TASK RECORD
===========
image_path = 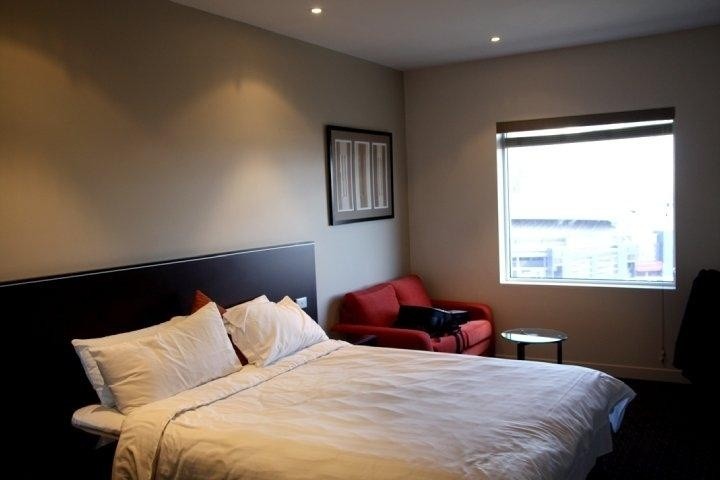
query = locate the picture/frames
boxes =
[324,123,394,227]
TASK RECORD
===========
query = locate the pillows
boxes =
[88,301,243,418]
[223,293,273,366]
[72,314,188,408]
[190,287,248,367]
[221,296,329,366]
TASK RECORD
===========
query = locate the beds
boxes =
[0,240,638,479]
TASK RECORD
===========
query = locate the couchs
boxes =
[330,272,497,357]
